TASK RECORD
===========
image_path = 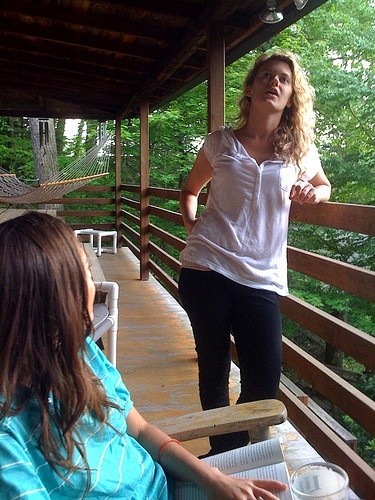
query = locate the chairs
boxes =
[91,281,119,369]
[148,398,287,444]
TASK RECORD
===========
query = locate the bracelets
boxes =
[158,439,181,465]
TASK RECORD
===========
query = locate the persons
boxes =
[177,47,331,458]
[0,211,285,500]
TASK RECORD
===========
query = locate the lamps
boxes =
[259,0,283,24]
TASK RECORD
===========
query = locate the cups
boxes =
[290,462,349,500]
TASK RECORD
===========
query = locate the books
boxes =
[174,438,294,500]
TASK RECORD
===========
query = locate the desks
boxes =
[74,228,117,256]
